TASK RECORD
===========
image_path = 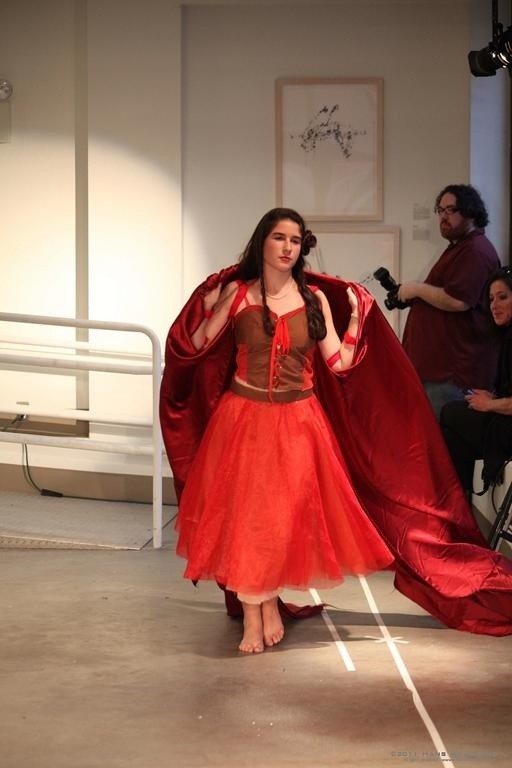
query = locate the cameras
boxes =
[372,266,414,310]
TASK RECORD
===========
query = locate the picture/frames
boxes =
[304,222,401,339]
[274,76,384,223]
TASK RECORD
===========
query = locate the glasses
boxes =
[434,206,459,216]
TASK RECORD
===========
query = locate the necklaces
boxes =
[258,278,294,299]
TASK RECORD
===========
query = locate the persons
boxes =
[395,182,503,425]
[171,209,396,655]
[437,267,510,512]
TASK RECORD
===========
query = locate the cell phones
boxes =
[461,386,476,396]
